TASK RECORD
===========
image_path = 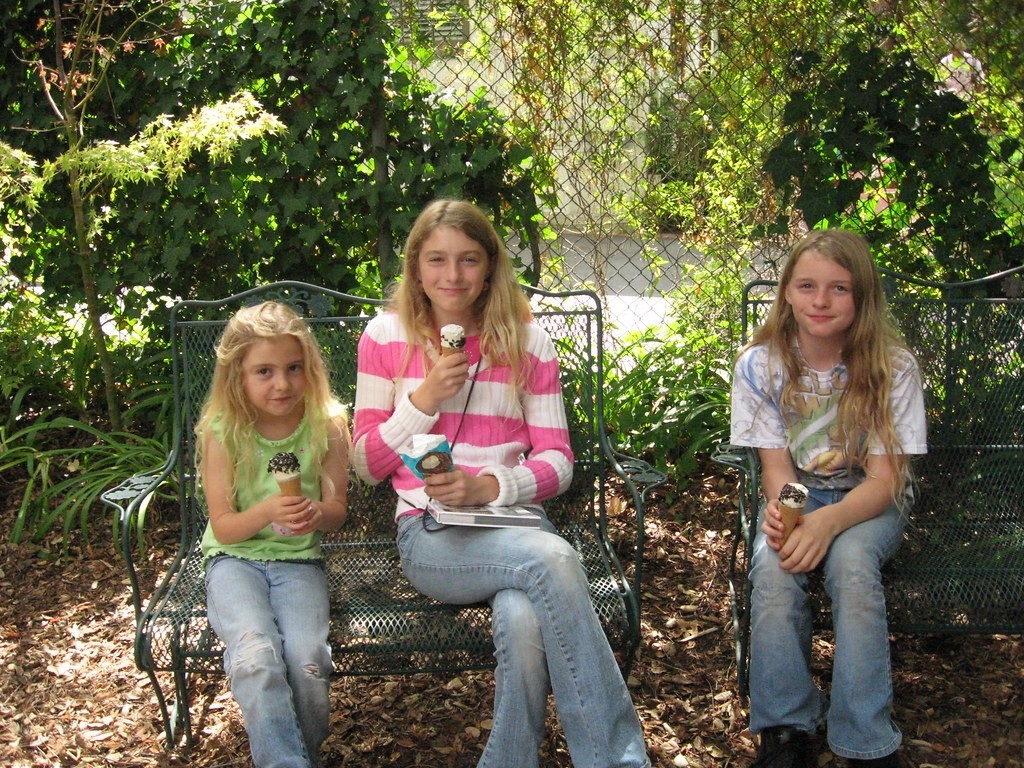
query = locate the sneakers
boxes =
[746,726,902,768]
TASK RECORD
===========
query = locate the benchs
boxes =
[727,267,1023,700]
[123,276,649,749]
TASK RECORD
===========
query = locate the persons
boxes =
[352,201,657,768]
[729,231,932,767]
[196,301,352,767]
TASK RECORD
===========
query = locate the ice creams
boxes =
[414,451,451,478]
[775,482,809,548]
[439,324,465,358]
[266,452,301,497]
